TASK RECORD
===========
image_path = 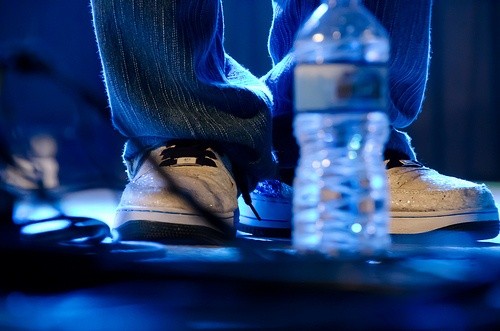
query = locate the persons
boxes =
[92,0,500,246]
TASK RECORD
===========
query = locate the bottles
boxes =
[291,0,393,261]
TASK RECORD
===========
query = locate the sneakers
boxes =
[111,137,263,246]
[236,125,500,244]
